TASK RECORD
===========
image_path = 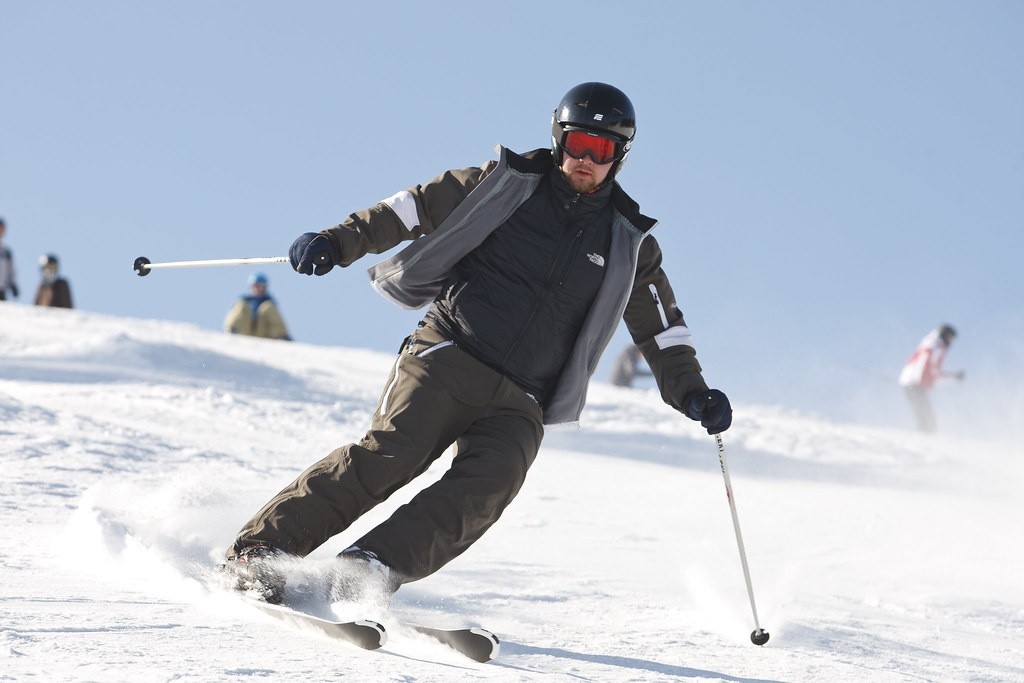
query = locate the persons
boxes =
[899,322,965,432]
[34,254,72,308]
[217,81,732,608]
[612,343,653,387]
[0,220,19,302]
[223,272,291,340]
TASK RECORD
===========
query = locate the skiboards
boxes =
[242,596,500,668]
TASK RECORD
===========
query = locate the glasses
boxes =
[559,127,627,165]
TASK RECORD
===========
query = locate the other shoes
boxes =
[218,544,286,604]
[323,546,403,616]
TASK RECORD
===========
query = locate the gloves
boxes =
[686,388,732,435]
[289,233,340,275]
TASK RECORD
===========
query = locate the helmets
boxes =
[38,254,58,271]
[551,82,637,179]
[249,273,267,284]
